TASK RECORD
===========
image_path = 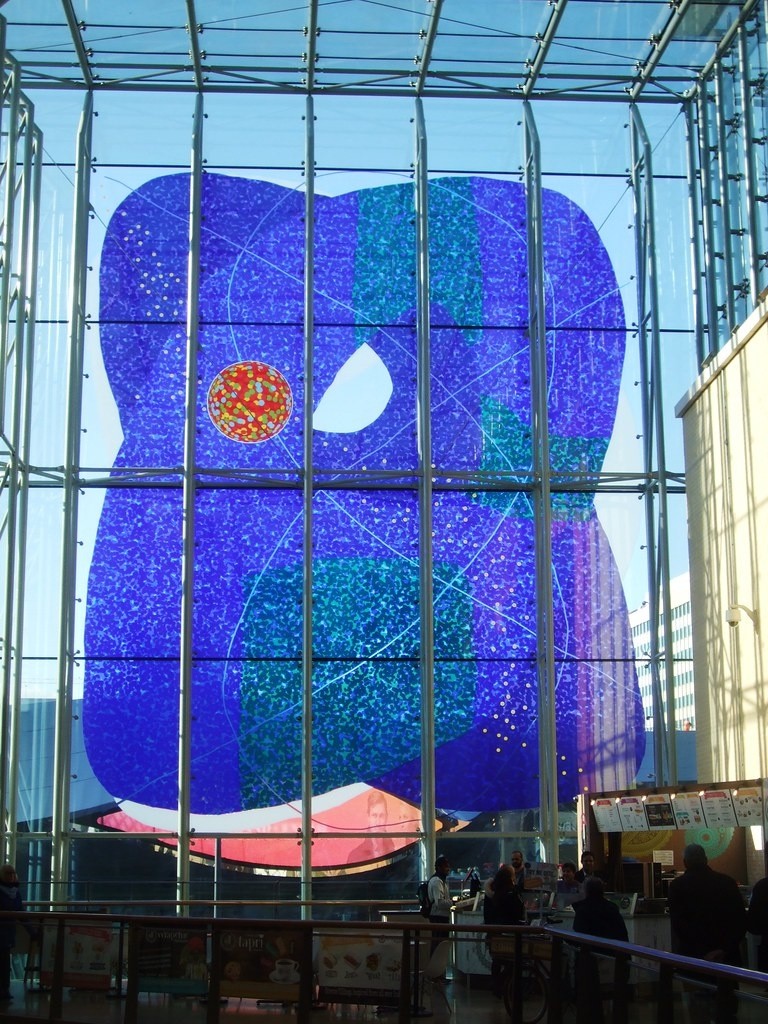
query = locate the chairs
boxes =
[410,940,453,1013]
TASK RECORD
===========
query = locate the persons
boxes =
[484,851,528,1001]
[557,851,633,1024]
[668,845,768,1023]
[1,867,38,1004]
[428,859,452,983]
[346,791,419,864]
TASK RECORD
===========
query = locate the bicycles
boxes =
[501,913,612,1023]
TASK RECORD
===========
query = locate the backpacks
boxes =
[417,875,443,918]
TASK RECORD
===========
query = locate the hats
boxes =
[435,857,448,866]
